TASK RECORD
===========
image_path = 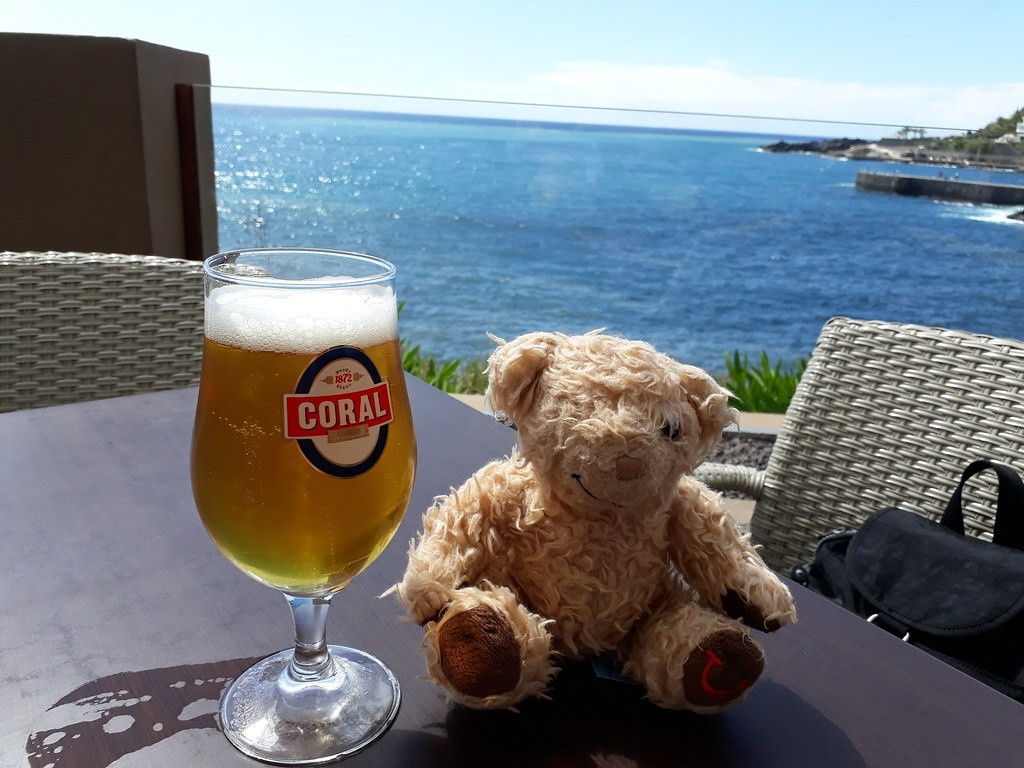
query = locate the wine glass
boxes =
[188,247,419,765]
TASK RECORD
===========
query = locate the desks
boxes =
[0,369,1024,768]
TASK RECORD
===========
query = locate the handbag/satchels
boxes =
[792,461,1024,704]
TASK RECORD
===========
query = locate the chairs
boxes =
[683,314,1024,579]
[0,252,270,414]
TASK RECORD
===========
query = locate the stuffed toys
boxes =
[394,327,799,716]
